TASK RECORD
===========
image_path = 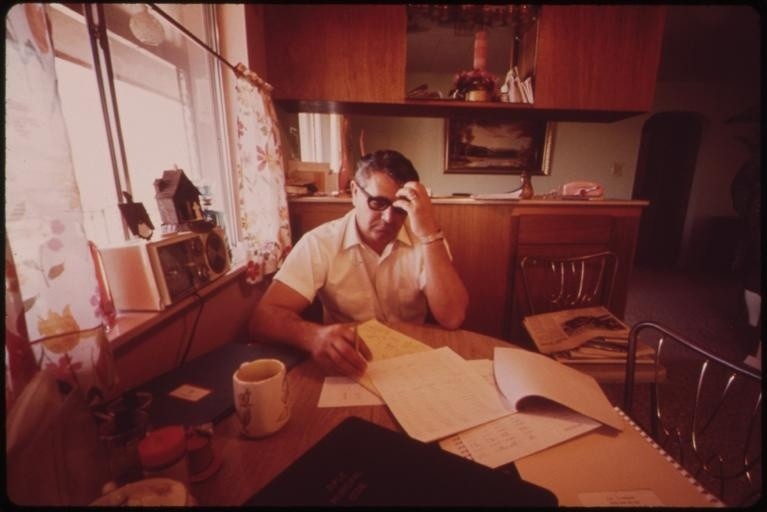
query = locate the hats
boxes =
[137,423,224,483]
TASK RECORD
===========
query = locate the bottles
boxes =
[520,168,534,199]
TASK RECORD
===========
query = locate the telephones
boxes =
[563,181,604,197]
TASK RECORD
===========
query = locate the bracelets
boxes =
[419,230,444,244]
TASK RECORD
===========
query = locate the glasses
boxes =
[354,181,407,215]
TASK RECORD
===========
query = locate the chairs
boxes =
[626,321,761,506]
[519,250,668,445]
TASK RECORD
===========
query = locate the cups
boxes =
[233,357,294,439]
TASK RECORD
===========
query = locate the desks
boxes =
[22,317,730,508]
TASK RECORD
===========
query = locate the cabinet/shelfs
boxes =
[705,217,752,276]
[282,191,651,342]
[254,5,664,113]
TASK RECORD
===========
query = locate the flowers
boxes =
[454,69,498,90]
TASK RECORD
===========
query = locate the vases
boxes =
[465,85,487,102]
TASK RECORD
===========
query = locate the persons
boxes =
[246,151,469,378]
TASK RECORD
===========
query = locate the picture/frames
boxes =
[441,118,558,176]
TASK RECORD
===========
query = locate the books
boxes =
[470,189,522,199]
[284,180,317,194]
[513,407,725,508]
[317,307,665,470]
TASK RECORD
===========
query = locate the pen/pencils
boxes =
[352,322,359,352]
